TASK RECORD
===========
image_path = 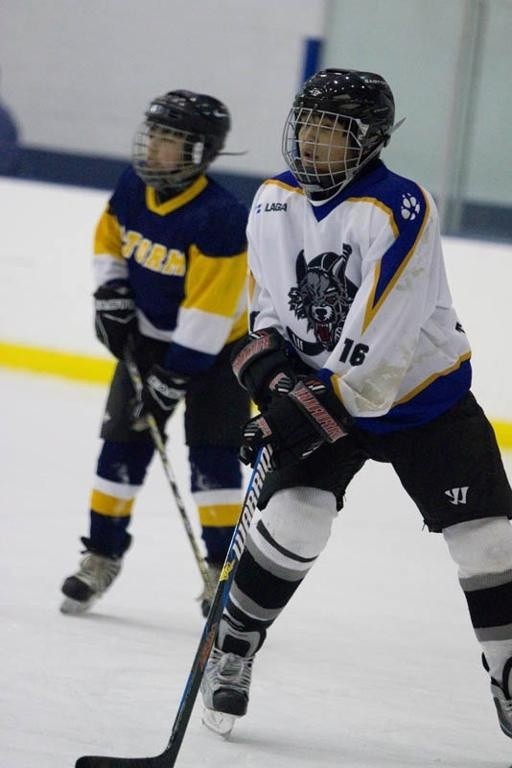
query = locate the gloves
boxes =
[94,284,140,358]
[229,326,349,468]
[125,366,190,443]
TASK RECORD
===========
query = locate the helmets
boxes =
[148,90,229,162]
[295,69,394,145]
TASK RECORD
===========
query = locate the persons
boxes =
[199,68,511,738]
[60,90,256,615]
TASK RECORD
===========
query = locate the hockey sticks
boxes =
[76,444,278,767]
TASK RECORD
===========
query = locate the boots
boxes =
[63,531,132,602]
[202,555,265,714]
[482,652,511,738]
